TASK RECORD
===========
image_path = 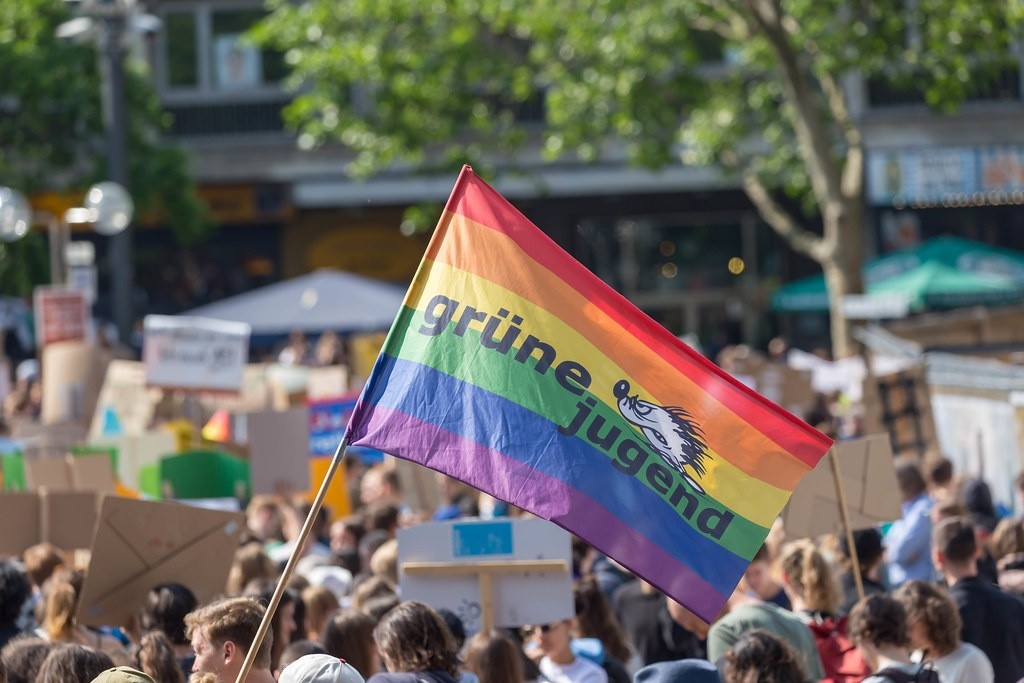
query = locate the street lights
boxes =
[54,9,164,355]
[0,180,133,288]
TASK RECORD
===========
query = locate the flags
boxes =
[345,163,838,626]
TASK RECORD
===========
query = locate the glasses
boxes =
[523,622,563,636]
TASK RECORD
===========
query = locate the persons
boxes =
[266,330,352,368]
[4,360,43,435]
[440,525,709,682]
[719,632,818,683]
[366,599,480,683]
[7,470,405,682]
[663,593,828,681]
[739,460,1023,683]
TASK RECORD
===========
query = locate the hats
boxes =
[91,666,154,683]
[279,654,365,682]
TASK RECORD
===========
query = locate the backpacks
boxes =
[878,667,940,682]
[799,618,871,683]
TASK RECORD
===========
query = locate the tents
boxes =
[774,234,1023,321]
[175,267,414,338]
